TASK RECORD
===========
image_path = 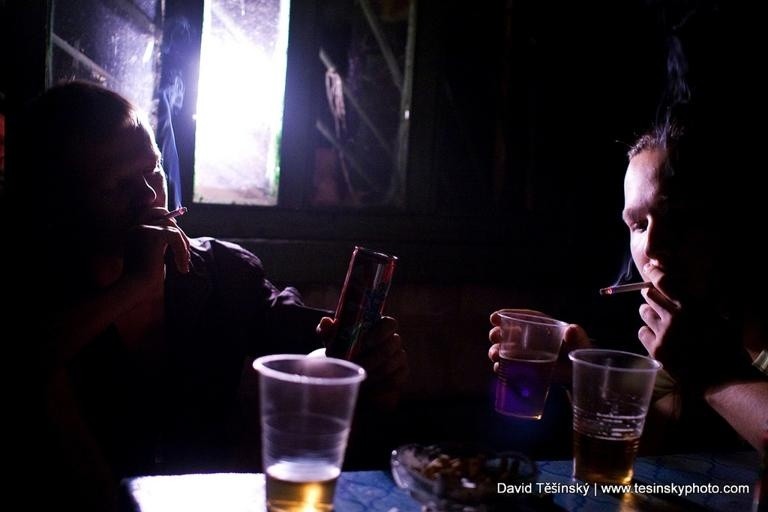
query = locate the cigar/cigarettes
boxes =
[599,281,653,295]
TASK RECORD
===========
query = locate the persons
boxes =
[0,81,410,512]
[159,207,187,219]
[487,124,768,457]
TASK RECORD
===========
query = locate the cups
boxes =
[495,311,663,488]
[253,354,368,512]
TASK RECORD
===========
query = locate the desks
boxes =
[118,453,760,512]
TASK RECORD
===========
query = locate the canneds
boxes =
[325,245,400,364]
